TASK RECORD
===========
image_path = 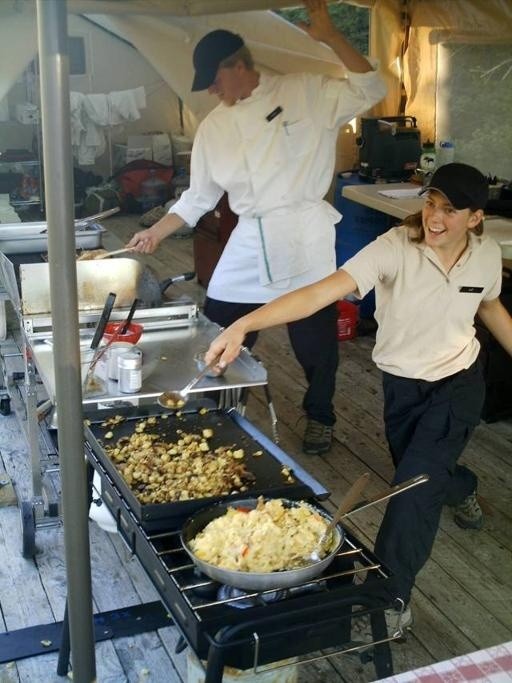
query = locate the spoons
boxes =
[285,473,372,572]
[156,349,223,409]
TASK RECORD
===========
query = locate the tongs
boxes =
[73,206,122,232]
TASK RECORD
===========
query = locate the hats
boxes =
[190,28,244,93]
[417,161,489,212]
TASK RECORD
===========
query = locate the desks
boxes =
[342,181,512,274]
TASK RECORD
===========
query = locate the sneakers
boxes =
[335,602,414,654]
[450,481,481,533]
[297,414,332,456]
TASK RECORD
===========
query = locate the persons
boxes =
[123,1,388,456]
[204,162,512,658]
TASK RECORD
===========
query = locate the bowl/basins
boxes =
[193,351,226,378]
[180,473,431,590]
[103,321,143,346]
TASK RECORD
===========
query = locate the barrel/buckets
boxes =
[335,300,361,342]
